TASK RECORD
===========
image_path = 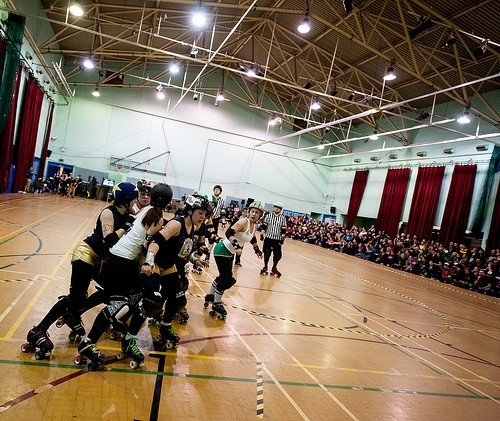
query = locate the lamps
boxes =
[341,0,353,12]
[91,88,100,97]
[446,34,456,46]
[310,95,321,110]
[457,108,471,124]
[98,69,103,76]
[382,63,397,82]
[297,14,311,34]
[302,80,355,101]
[118,73,123,80]
[369,128,380,141]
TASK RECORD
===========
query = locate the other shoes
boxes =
[234,261,242,267]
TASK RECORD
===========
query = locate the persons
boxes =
[23,166,99,199]
[108,179,264,369]
[72,183,173,371]
[260,203,286,277]
[22,182,138,360]
[284,213,500,298]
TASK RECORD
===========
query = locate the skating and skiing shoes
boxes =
[74,337,106,371]
[173,308,190,324]
[270,267,282,278]
[116,337,145,369]
[55,311,86,341]
[260,267,269,274]
[191,265,203,275]
[147,311,164,329]
[209,301,227,321]
[106,319,129,341]
[20,325,54,360]
[155,323,181,350]
[204,294,215,308]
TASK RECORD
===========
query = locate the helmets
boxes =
[112,182,139,199]
[151,183,173,203]
[213,185,222,192]
[186,194,210,211]
[136,179,153,193]
[274,202,283,210]
[248,201,265,217]
